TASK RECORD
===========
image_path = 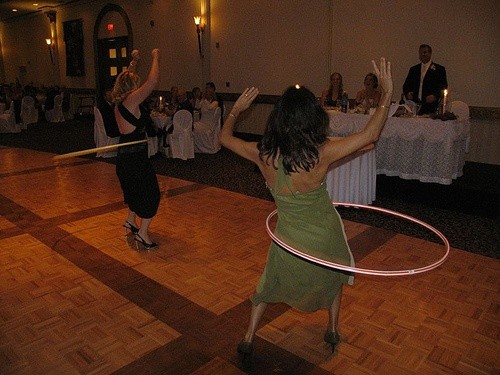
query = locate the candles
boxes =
[443,90,447,114]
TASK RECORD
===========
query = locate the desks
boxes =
[323,136,377,205]
[327,110,468,185]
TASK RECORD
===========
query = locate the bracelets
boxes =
[377,104,390,109]
[228,113,238,120]
[129,61,137,67]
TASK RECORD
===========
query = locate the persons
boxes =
[220,57,393,361]
[98,82,218,158]
[402,44,447,115]
[321,72,382,110]
[0,82,69,123]
[111,49,160,248]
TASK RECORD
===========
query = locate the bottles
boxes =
[342,92,348,112]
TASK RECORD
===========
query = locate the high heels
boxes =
[122,221,140,235]
[133,233,159,253]
[324,331,339,353]
[237,340,253,363]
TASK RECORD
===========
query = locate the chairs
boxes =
[74,96,95,146]
[146,135,158,158]
[449,102,469,118]
[0,92,76,133]
[194,107,221,155]
[93,105,120,158]
[166,110,196,161]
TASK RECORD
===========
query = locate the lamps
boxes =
[193,16,202,26]
[45,38,52,45]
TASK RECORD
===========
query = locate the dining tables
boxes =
[150,111,200,148]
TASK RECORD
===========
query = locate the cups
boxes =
[336,100,342,111]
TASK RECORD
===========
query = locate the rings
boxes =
[245,94,249,97]
